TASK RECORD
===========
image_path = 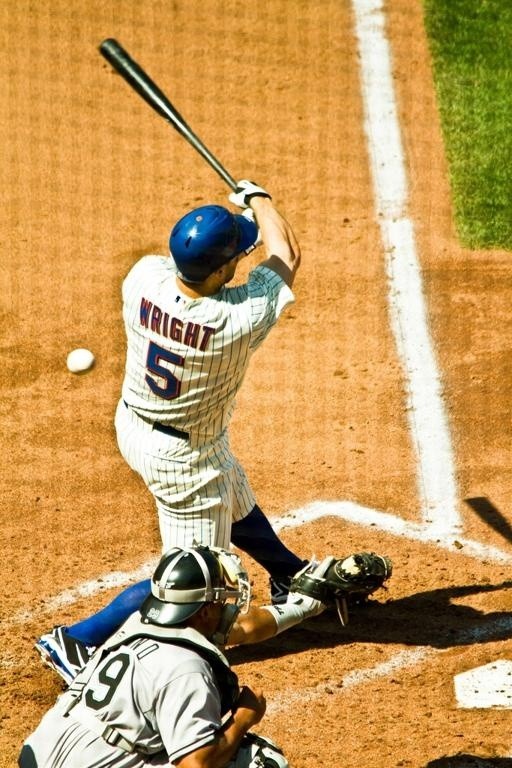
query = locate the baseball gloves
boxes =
[289,551,393,622]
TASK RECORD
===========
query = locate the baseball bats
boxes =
[98,38,242,194]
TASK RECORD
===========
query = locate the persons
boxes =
[34,179,315,686]
[14,540,289,768]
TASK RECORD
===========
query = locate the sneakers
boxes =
[269,560,309,605]
[33,624,97,691]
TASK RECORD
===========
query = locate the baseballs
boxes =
[67,350,94,374]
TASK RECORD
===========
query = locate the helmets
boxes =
[169,204,257,281]
[139,544,252,650]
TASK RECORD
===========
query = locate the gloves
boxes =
[259,556,336,638]
[229,180,271,255]
